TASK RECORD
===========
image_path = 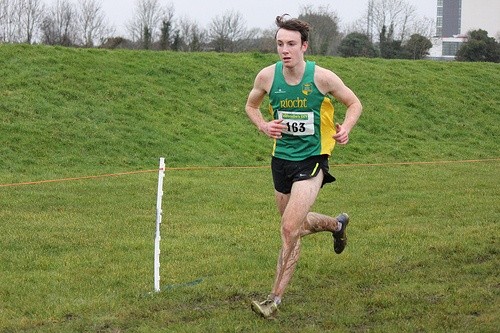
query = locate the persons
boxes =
[246,13,365,318]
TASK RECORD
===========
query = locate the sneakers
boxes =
[333,213,350,254]
[251,300,280,319]
